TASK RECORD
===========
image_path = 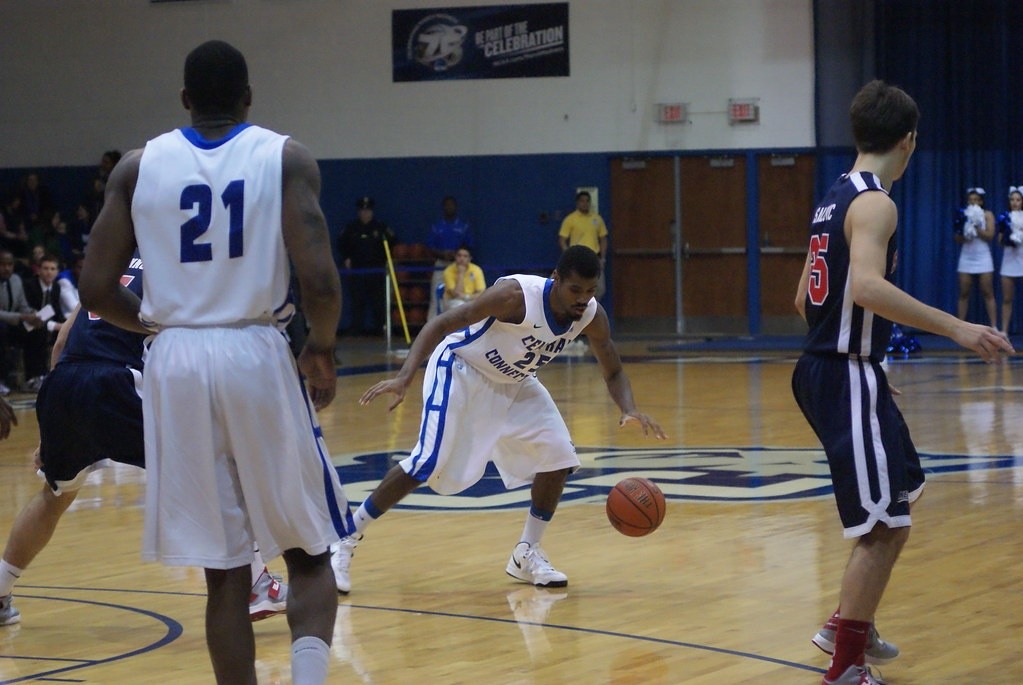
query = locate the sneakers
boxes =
[821,662,888,685]
[506,589,568,620]
[505,541,567,586]
[0,592,21,625]
[249,566,290,621]
[331,601,355,657]
[328,532,364,592]
[811,616,900,664]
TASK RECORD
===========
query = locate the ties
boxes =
[45,289,50,305]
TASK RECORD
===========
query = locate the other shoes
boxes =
[0,384,10,393]
[22,377,42,392]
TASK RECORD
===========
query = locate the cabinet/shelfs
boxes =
[387,256,438,328]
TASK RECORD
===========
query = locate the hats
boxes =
[356,197,375,210]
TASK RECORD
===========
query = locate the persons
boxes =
[558,191,608,269]
[791,80,1016,685]
[337,196,398,330]
[997,186,1023,333]
[954,187,997,327]
[330,245,667,595]
[0,247,288,625]
[0,151,121,395]
[78,40,356,684]
[443,246,486,312]
[427,196,476,322]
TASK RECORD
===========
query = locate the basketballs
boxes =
[606,476,666,538]
